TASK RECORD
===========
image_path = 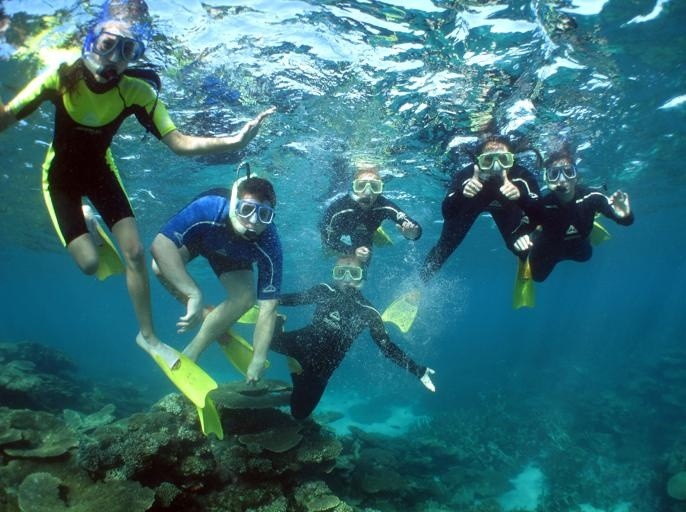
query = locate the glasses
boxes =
[477,151,515,170]
[90,31,146,61]
[237,200,274,225]
[546,164,576,181]
[352,179,384,194]
[332,266,363,280]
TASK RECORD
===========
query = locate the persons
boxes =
[317,168,424,263]
[268,254,437,422]
[417,136,635,284]
